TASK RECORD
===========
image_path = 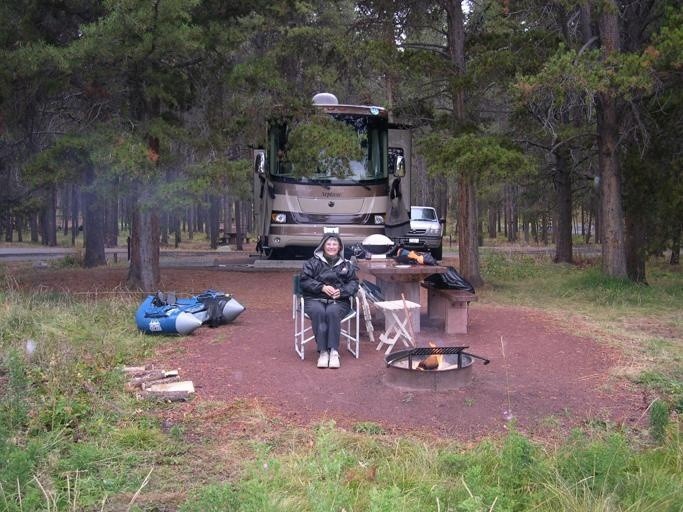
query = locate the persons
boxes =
[299,232,358,368]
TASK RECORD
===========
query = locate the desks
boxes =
[357,258,448,334]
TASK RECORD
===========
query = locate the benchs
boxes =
[420,281,478,334]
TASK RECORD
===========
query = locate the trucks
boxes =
[251,91,415,261]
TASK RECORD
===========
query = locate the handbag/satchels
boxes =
[424,266,474,292]
[394,249,436,265]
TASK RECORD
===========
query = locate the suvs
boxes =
[391,206,446,261]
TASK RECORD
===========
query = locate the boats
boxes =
[133,289,248,338]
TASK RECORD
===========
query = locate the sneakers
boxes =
[317,352,328,367]
[329,352,340,367]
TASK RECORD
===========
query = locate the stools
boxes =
[374,299,422,357]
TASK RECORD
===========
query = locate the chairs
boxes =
[292,273,360,359]
